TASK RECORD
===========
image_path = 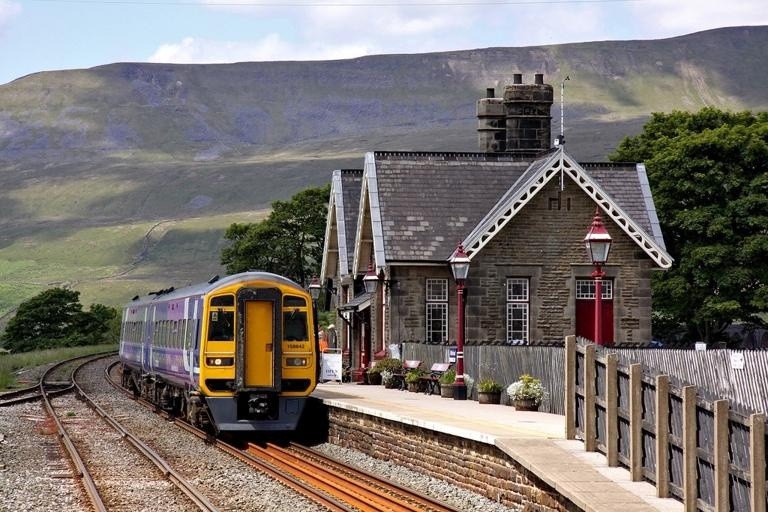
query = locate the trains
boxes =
[117,269,324,445]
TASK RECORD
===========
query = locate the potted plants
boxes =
[365,359,381,385]
[438,368,456,398]
[475,378,504,404]
[404,369,425,392]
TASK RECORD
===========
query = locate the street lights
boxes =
[585,202,612,346]
[307,274,322,384]
[447,238,473,384]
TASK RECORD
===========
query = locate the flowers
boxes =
[506,375,550,407]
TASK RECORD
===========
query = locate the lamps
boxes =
[362,256,400,295]
[308,272,337,312]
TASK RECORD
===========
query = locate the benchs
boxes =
[419,363,452,395]
[392,359,424,391]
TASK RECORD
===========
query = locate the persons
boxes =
[316,323,338,353]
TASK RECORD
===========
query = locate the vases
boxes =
[513,398,539,411]
